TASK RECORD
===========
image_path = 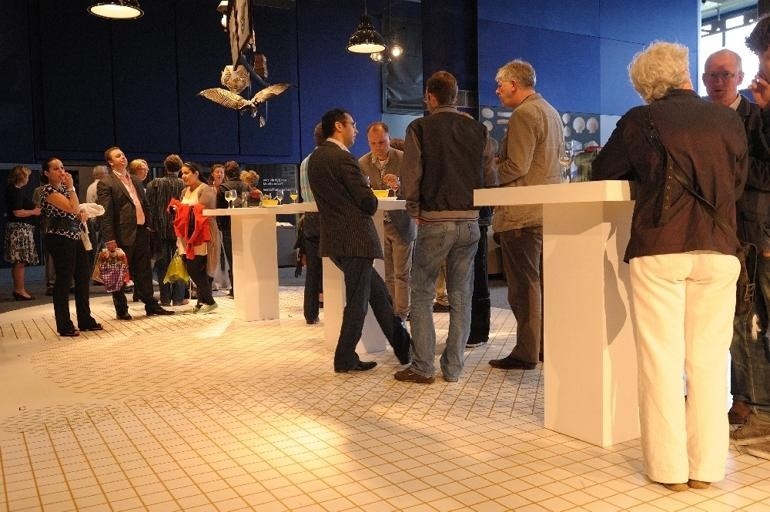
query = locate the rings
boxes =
[757,79,759,83]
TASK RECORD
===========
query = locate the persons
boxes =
[745,15,770,464]
[3,146,262,337]
[703,48,770,437]
[390,139,406,151]
[394,71,492,384]
[590,40,749,493]
[459,111,500,348]
[485,58,566,370]
[307,107,426,373]
[299,122,325,324]
[356,122,419,321]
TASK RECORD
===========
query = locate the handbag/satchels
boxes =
[99,260,128,293]
[91,248,128,286]
[737,240,758,316]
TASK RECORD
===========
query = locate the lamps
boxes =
[87,0,144,19]
[346,0,386,54]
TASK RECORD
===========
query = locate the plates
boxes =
[378,196,396,201]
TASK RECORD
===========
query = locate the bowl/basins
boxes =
[373,189,390,198]
[262,200,278,208]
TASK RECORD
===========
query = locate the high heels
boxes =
[14,292,37,301]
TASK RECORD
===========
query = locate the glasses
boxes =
[342,121,356,128]
[184,162,196,170]
[706,72,734,80]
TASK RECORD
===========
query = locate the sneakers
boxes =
[433,302,449,312]
[662,484,688,492]
[743,443,770,460]
[728,401,749,424]
[688,481,710,489]
[182,307,199,315]
[446,378,458,382]
[466,341,485,347]
[395,368,435,383]
[197,303,218,314]
[729,412,770,445]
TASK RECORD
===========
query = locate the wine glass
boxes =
[389,176,400,200]
[290,188,299,203]
[276,189,285,205]
[559,140,575,183]
[230,189,238,209]
[225,191,232,208]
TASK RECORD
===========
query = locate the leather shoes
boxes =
[60,331,80,336]
[539,353,543,361]
[489,355,535,369]
[147,307,175,316]
[163,303,170,306]
[117,313,131,320]
[307,317,319,324]
[45,287,53,296]
[399,355,410,364]
[334,362,377,372]
[173,300,188,305]
[80,323,102,331]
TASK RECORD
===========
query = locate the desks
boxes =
[203,204,299,321]
[297,200,406,354]
[473,180,635,448]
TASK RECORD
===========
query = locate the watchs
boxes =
[396,176,401,182]
